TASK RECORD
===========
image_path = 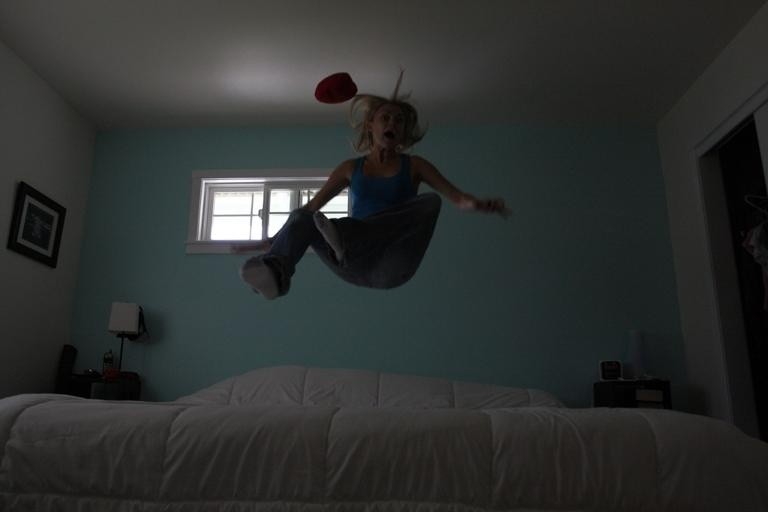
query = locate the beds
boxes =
[0,393,768,512]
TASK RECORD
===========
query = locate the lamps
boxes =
[107,302,139,372]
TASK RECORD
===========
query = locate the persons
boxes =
[228,66,518,301]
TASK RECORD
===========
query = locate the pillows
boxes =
[184,364,567,409]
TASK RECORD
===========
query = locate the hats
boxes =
[315,71,358,104]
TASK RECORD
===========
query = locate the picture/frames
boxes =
[6,181,67,268]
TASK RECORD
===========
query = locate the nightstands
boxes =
[54,370,141,401]
[593,380,673,410]
